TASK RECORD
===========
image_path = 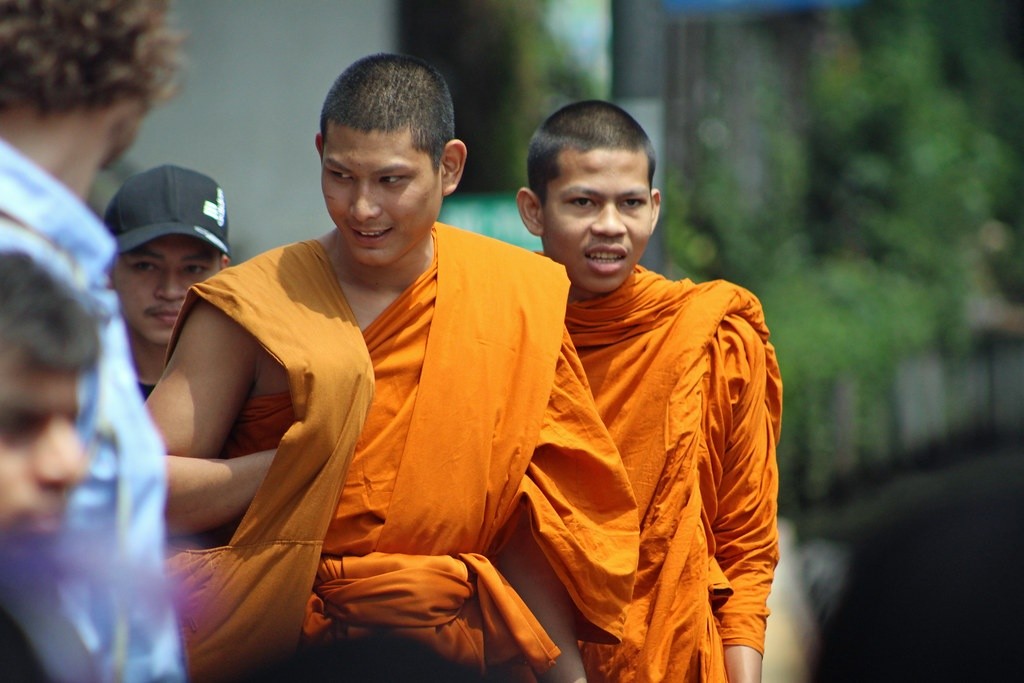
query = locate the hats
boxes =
[104,164,230,256]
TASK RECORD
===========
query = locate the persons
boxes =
[145,53,784,682]
[0,1,233,683]
